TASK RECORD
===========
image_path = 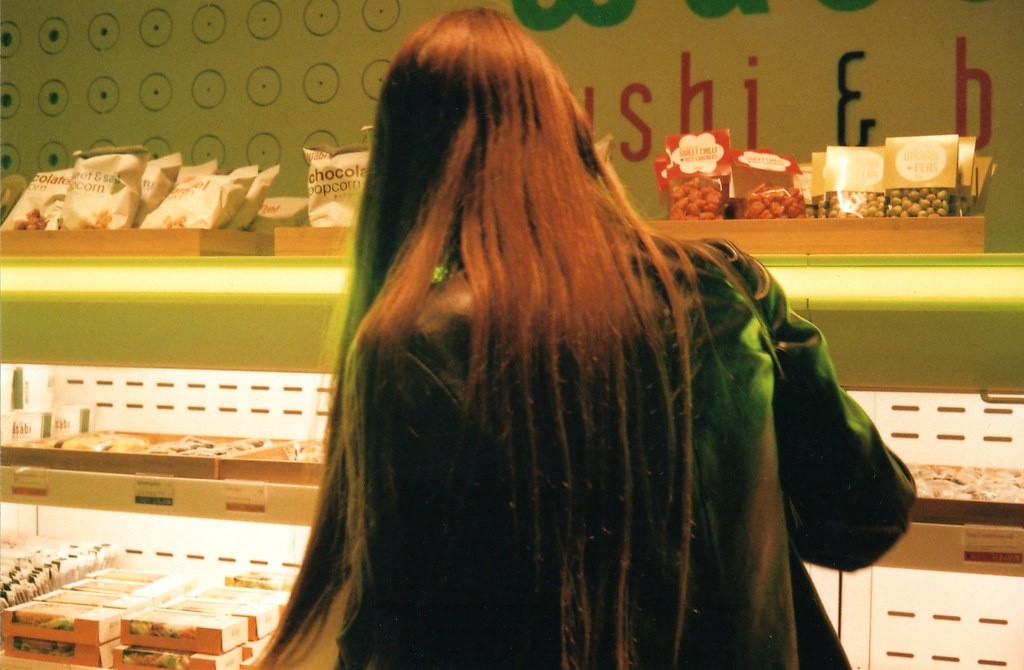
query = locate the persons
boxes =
[256,7,917,670]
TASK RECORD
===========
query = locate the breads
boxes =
[905,464,1024,505]
[21,431,324,463]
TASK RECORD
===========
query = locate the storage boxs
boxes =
[0,568,291,670]
